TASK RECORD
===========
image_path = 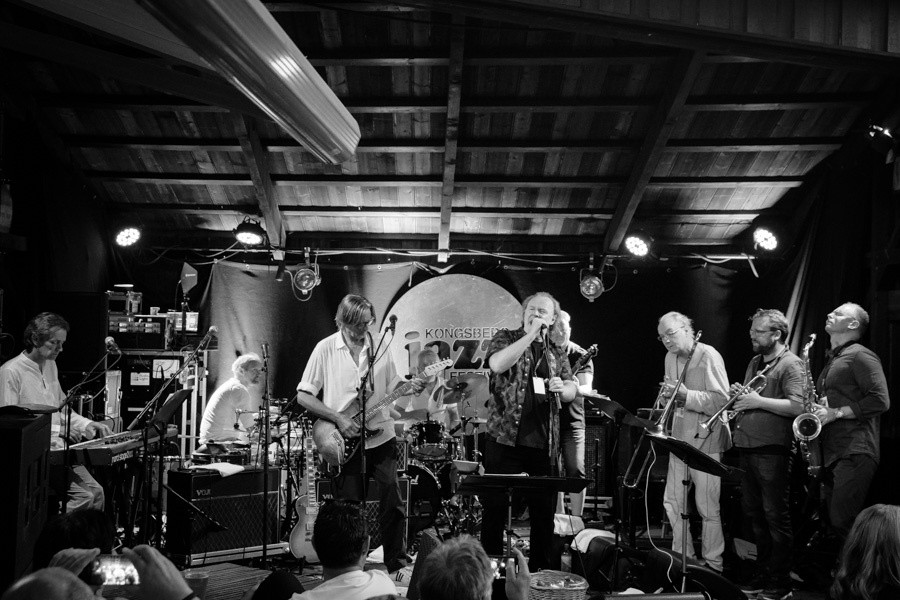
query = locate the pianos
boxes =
[51,427,179,554]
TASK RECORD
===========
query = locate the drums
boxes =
[419,437,460,464]
[398,459,443,533]
[277,448,306,469]
[284,428,303,448]
[408,419,449,457]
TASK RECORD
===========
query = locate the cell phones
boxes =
[85,553,141,586]
[486,555,517,580]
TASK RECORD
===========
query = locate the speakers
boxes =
[1,408,53,596]
[569,528,649,596]
[584,410,615,498]
[638,546,748,600]
[168,466,281,562]
[312,476,411,555]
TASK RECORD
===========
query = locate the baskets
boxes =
[529,570,589,600]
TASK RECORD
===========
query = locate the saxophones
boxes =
[793,334,822,478]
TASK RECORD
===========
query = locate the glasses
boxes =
[355,318,374,330]
[657,330,681,342]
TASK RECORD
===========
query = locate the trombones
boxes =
[622,330,703,489]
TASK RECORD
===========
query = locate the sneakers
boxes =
[390,568,412,586]
[741,578,763,592]
[757,582,793,600]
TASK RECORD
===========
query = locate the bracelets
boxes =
[183,592,197,600]
[836,408,843,418]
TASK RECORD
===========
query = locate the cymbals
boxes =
[455,418,487,427]
[433,373,488,405]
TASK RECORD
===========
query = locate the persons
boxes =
[0,311,116,515]
[830,504,900,600]
[417,533,530,600]
[480,291,576,574]
[393,349,459,434]
[251,502,399,600]
[803,302,890,580]
[99,567,134,584]
[550,311,594,516]
[199,352,278,462]
[296,295,428,586]
[728,310,807,600]
[0,544,201,600]
[657,311,731,575]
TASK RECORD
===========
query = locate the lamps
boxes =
[625,227,656,257]
[112,222,142,247]
[579,266,605,303]
[294,262,318,295]
[236,221,263,245]
[752,224,784,253]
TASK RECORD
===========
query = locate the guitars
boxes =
[571,344,598,376]
[289,417,326,563]
[312,356,453,468]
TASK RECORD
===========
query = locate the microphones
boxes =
[200,326,218,346]
[389,314,397,336]
[260,339,270,361]
[529,318,548,329]
[105,336,121,354]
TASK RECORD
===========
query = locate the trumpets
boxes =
[699,364,771,433]
